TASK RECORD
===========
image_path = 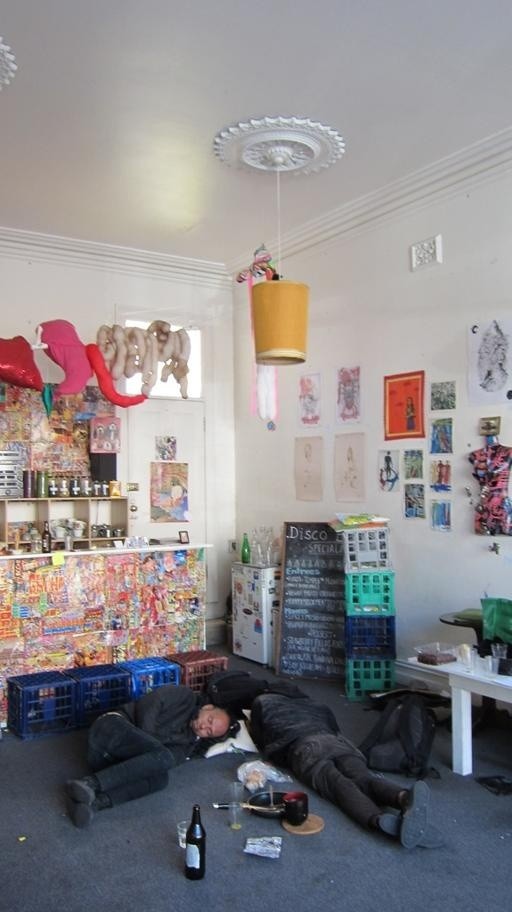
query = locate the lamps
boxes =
[214,115,346,365]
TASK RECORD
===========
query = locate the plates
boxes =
[282,813,326,835]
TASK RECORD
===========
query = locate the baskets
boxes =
[344,569,397,618]
[163,649,229,694]
[345,614,398,661]
[343,526,393,574]
[115,657,182,704]
[6,670,80,740]
[62,663,133,730]
[344,657,397,704]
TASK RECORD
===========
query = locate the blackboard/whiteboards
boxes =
[273,522,346,682]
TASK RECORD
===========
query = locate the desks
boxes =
[408,648,512,776]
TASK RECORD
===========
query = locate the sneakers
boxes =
[66,796,98,829]
[64,778,97,806]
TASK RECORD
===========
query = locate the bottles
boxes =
[42,521,51,553]
[241,533,250,563]
[23,470,121,498]
[185,805,207,880]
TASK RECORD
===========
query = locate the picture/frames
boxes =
[384,371,426,442]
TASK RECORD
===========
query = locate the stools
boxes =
[440,611,512,738]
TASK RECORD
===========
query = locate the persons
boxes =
[385,468,399,491]
[403,397,417,432]
[443,460,451,485]
[435,460,444,484]
[248,677,449,850]
[61,684,235,830]
[380,469,385,489]
[467,415,512,538]
[382,451,394,479]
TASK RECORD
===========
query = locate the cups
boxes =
[31,539,42,553]
[56,521,150,551]
[459,643,508,678]
[177,782,244,848]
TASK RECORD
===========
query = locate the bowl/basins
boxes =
[282,791,309,826]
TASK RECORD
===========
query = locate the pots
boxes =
[212,790,291,818]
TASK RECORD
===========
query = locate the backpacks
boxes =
[203,663,271,709]
[357,692,441,783]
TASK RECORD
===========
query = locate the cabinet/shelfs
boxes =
[0,497,130,553]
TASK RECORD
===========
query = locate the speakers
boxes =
[90,452,116,481]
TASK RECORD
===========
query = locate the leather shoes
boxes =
[398,778,432,851]
[376,812,450,849]
